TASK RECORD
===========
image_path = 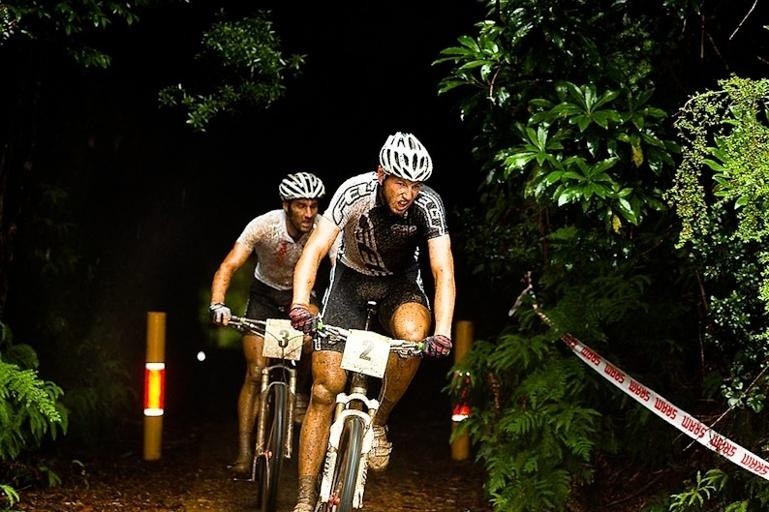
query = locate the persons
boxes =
[207,170,342,476]
[288,126,461,512]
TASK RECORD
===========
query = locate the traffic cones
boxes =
[452,321,473,461]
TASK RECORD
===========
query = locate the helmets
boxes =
[279,172,326,199]
[380,132,433,182]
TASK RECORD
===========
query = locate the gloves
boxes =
[423,336,453,358]
[291,304,312,332]
[208,302,231,327]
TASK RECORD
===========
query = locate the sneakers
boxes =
[367,408,392,472]
[294,493,316,512]
[234,454,252,474]
[292,392,309,422]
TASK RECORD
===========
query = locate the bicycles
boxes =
[227,314,304,512]
[308,323,425,512]
